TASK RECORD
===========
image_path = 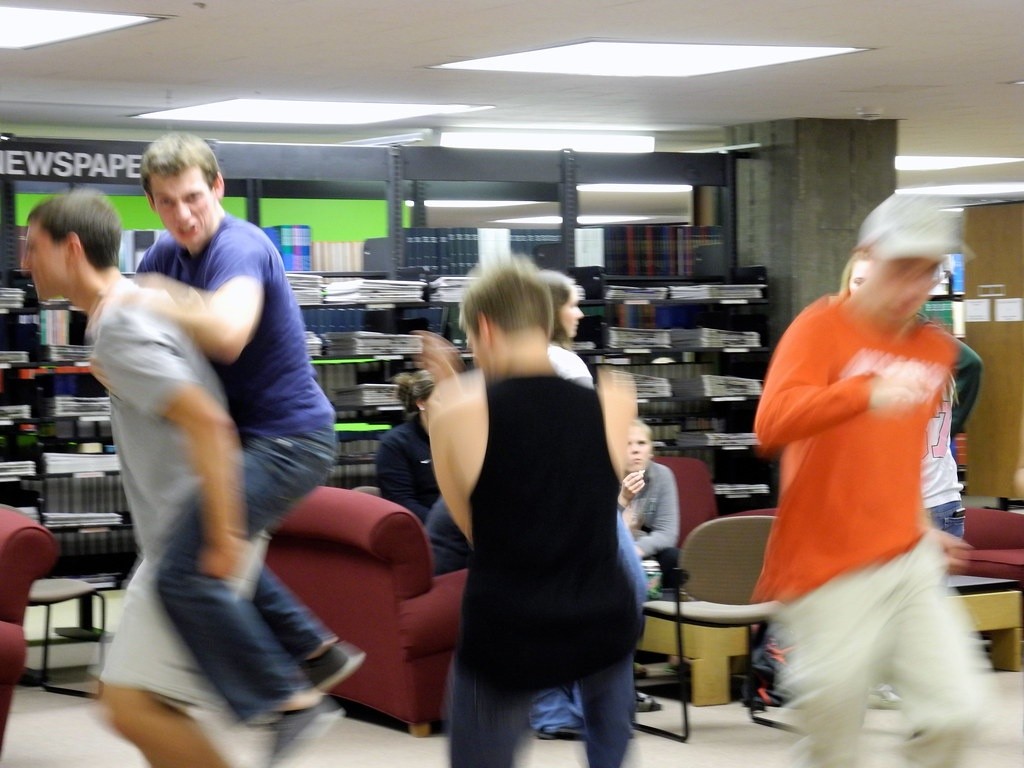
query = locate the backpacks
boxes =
[742,622,796,711]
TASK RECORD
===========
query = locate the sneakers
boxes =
[299,639,366,692]
[266,695,345,768]
[867,684,902,709]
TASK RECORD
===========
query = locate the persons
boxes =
[21,133,367,768]
[377,253,690,768]
[755,194,1001,768]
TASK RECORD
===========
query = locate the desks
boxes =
[634,572,1023,707]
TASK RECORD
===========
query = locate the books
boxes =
[0,225,770,527]
[919,253,966,468]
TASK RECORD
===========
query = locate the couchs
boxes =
[648,455,716,549]
[263,485,470,738]
[734,507,1024,581]
[0,508,59,755]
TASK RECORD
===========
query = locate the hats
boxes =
[858,193,954,262]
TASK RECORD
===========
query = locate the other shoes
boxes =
[538,725,587,739]
[636,691,655,712]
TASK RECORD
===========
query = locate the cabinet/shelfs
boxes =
[0,266,776,591]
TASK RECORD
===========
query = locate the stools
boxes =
[27,578,106,700]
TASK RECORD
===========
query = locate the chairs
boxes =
[632,516,813,741]
